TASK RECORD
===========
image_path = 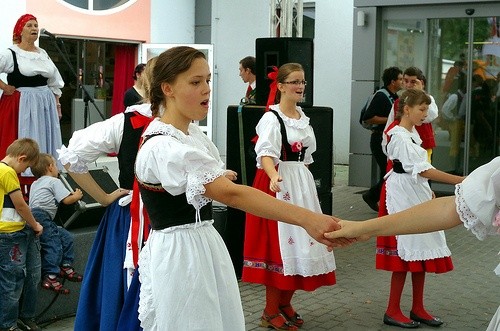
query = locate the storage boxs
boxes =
[54,168,125,229]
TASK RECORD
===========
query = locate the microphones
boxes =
[40,29,57,39]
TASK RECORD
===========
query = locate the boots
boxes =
[362,184,381,211]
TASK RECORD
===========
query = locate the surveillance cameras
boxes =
[465,9,474,15]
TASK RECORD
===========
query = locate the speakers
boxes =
[256,37,313,108]
[230,104,334,215]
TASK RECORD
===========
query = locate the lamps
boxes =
[358,10,367,26]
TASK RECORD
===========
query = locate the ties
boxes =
[246,85,252,97]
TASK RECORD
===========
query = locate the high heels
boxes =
[261,308,298,331]
[279,304,304,327]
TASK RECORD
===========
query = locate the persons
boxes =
[0,137,44,331]
[130,47,357,331]
[437,54,500,176]
[241,63,337,331]
[239,56,256,103]
[0,14,65,195]
[58,57,237,331]
[323,155,500,331]
[28,153,83,292]
[123,64,149,111]
[374,89,467,329]
[364,66,439,212]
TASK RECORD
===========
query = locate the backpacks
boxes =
[359,87,380,130]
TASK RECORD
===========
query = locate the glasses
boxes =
[282,81,308,85]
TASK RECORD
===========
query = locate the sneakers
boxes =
[58,264,83,282]
[42,274,70,294]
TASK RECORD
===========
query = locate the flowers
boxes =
[291,141,304,152]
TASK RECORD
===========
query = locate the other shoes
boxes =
[0,327,23,331]
[17,315,42,331]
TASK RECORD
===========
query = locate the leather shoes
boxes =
[409,311,443,326]
[384,312,420,328]
